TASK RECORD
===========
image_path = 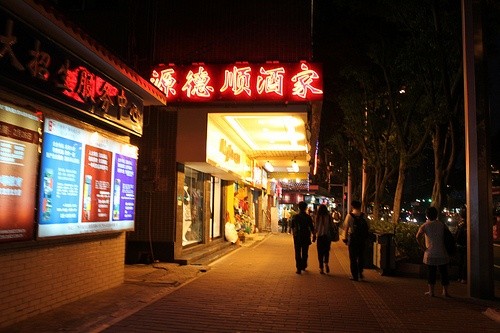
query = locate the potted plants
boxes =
[393,222,423,272]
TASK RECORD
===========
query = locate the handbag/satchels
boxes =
[328,216,338,241]
[443,223,456,254]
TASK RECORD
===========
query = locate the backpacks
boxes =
[349,212,369,240]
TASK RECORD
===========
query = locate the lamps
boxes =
[292,160,300,172]
[264,160,274,172]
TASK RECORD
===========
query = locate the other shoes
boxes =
[302,267,305,270]
[350,277,357,281]
[320,271,323,273]
[424,291,433,296]
[443,293,448,296]
[296,271,301,273]
[325,265,329,272]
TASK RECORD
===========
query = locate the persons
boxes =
[343,201,368,283]
[292,201,316,274]
[282,206,288,233]
[281,201,449,299]
[289,207,297,233]
[316,204,336,274]
[415,206,450,296]
